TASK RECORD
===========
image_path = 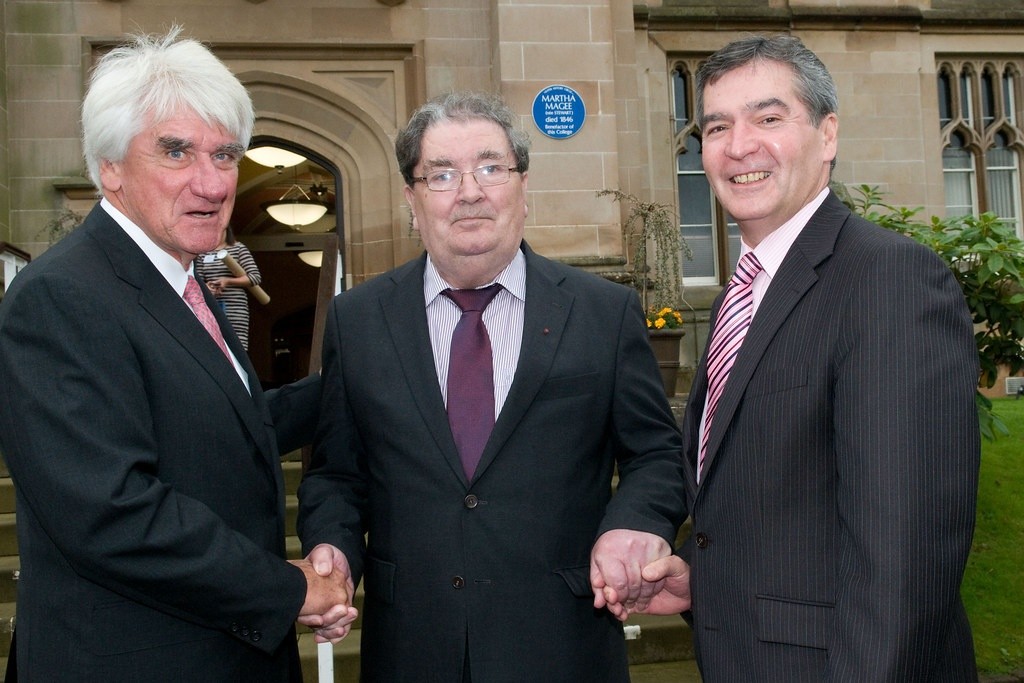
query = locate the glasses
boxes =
[412,164,522,191]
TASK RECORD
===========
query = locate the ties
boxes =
[438,283,504,485]
[183,275,234,367]
[700,251,764,481]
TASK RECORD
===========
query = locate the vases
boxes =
[646,328,686,398]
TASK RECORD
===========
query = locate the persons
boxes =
[294,89,690,682]
[197,221,263,351]
[604,29,985,683]
[0,38,359,683]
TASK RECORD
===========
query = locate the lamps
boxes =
[242,144,341,270]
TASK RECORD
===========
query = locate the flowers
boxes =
[644,305,686,328]
